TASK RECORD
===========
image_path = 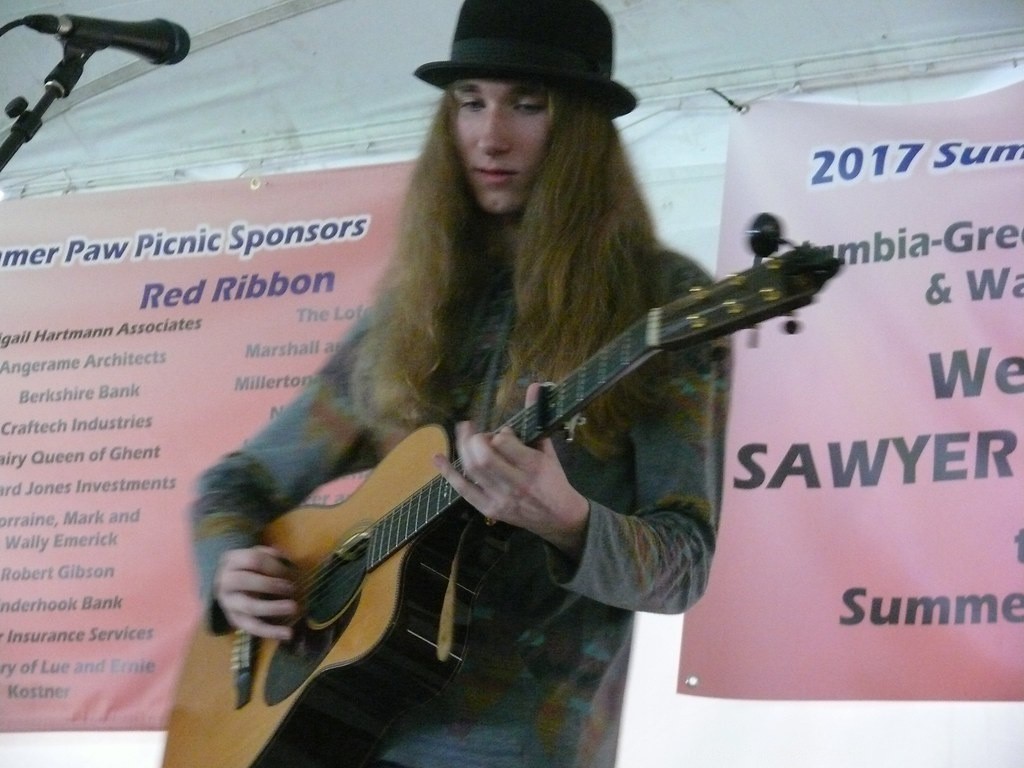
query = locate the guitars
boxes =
[157,212,840,767]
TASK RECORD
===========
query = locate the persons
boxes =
[188,0,732,767]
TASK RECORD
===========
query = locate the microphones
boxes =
[21,13,190,66]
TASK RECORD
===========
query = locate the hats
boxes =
[416,0,636,121]
